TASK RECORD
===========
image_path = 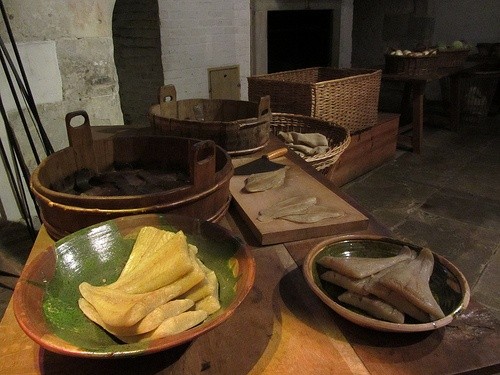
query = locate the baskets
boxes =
[383,40,473,75]
[269,110,351,176]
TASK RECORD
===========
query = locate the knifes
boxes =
[234,148,288,175]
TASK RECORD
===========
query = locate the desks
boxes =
[0,123,500,375]
[382,62,481,154]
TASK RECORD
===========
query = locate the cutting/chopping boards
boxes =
[227,153,370,245]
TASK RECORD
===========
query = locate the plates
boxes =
[13,213,257,359]
[303,234,471,333]
